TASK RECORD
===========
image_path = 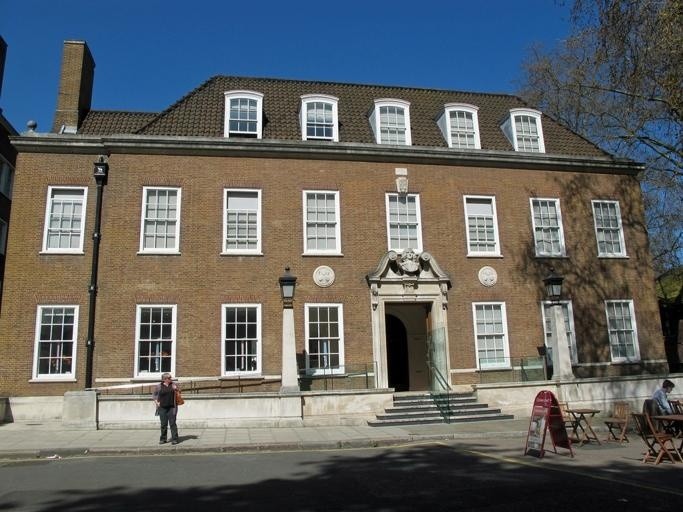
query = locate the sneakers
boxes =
[159,439,177,444]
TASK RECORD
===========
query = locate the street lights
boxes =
[541,265,577,384]
[275,263,300,395]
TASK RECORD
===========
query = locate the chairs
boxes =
[558,399,683,465]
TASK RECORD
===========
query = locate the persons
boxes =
[150,373,180,444]
[400,246,419,272]
[651,378,682,438]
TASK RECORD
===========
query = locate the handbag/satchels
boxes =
[176,390,184,405]
